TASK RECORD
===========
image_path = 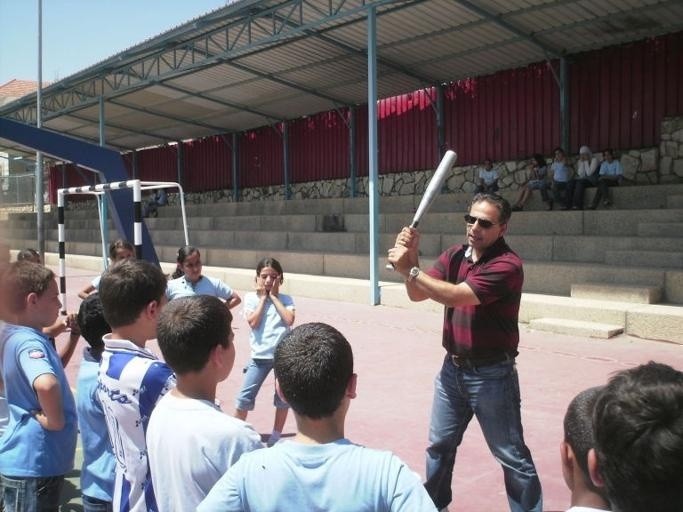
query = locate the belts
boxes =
[445,346,519,368]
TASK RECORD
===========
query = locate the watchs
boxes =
[408,267,420,281]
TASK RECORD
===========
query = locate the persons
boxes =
[473,158,499,196]
[146,293,264,511]
[142,188,167,218]
[561,361,682,510]
[165,246,242,309]
[2,239,176,511]
[510,147,623,211]
[232,256,295,447]
[196,323,438,512]
[387,194,544,511]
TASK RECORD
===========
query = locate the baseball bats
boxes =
[386,149,458,273]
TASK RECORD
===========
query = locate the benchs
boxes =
[0,176,682,345]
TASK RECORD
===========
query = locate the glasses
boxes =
[463,215,502,230]
